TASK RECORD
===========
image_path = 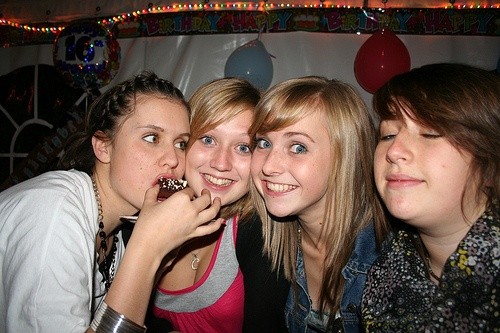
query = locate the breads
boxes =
[155,177,188,199]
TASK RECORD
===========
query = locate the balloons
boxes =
[223,40,274,93]
[354,28,411,94]
[52,20,123,92]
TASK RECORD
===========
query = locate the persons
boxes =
[143,76,291,333]
[248,76,394,333]
[361,63,500,333]
[0,70,226,333]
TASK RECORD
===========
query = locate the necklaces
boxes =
[185,238,205,269]
[89,173,118,292]
[426,255,441,282]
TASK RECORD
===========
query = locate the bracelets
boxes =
[90,300,147,333]
